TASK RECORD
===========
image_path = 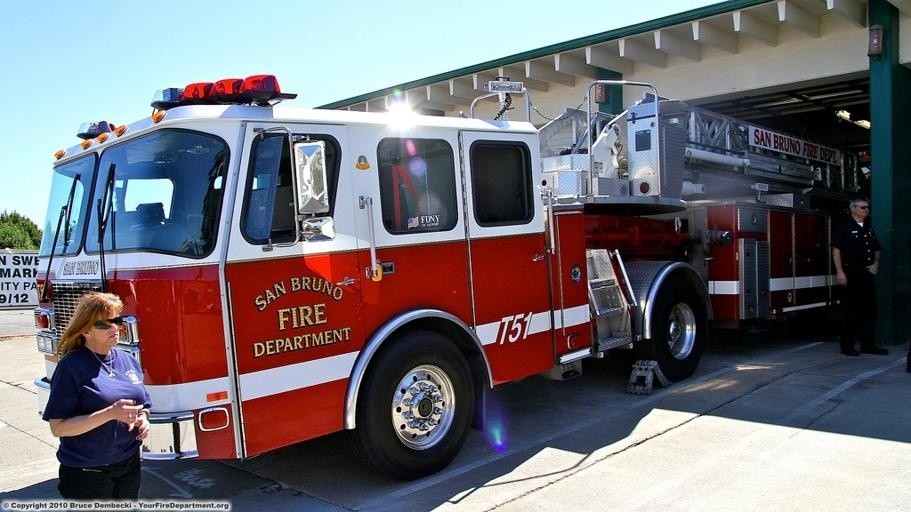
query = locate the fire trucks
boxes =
[33,78,868,482]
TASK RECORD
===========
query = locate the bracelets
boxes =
[139,408,151,419]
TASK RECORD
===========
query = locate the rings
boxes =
[129,414,133,418]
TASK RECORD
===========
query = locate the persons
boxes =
[41,291,154,499]
[830,195,890,357]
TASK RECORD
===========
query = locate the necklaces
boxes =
[86,345,116,378]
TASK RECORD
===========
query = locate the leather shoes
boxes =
[840,343,888,357]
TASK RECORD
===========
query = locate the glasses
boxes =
[852,205,870,209]
[94,317,123,329]
